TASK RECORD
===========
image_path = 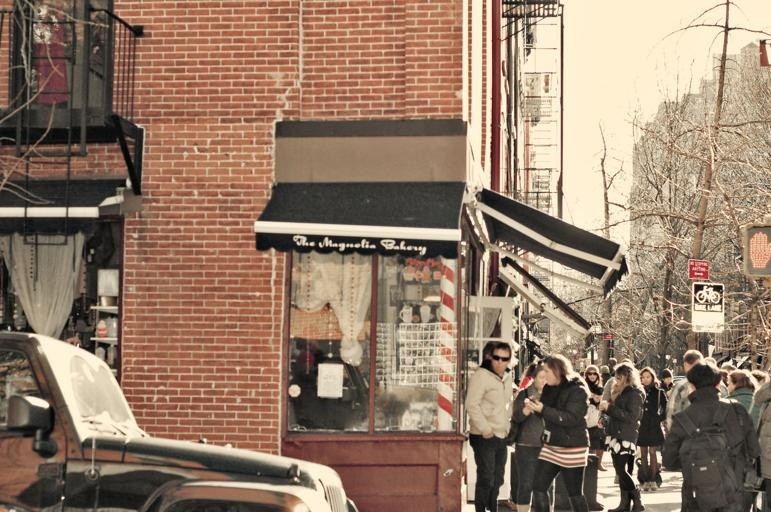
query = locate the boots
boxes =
[533,491,549,512]
[607,489,643,512]
[570,492,604,512]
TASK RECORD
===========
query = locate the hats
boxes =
[600,366,610,374]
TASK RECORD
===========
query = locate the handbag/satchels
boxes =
[507,419,519,446]
[637,469,662,485]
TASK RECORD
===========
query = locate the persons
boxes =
[510,366,555,511]
[664,349,730,432]
[597,363,647,512]
[517,355,770,483]
[523,354,590,511]
[639,367,668,491]
[662,364,761,512]
[464,340,515,512]
[749,367,771,512]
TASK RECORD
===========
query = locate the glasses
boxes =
[585,371,597,376]
[490,354,512,364]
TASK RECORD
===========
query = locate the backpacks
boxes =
[672,398,740,509]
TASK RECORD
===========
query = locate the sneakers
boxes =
[597,464,620,483]
[639,482,659,491]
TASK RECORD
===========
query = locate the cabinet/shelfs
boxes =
[89,306,119,376]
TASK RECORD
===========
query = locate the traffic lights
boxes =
[744,223,771,278]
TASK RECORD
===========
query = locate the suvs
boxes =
[0,331,358,512]
